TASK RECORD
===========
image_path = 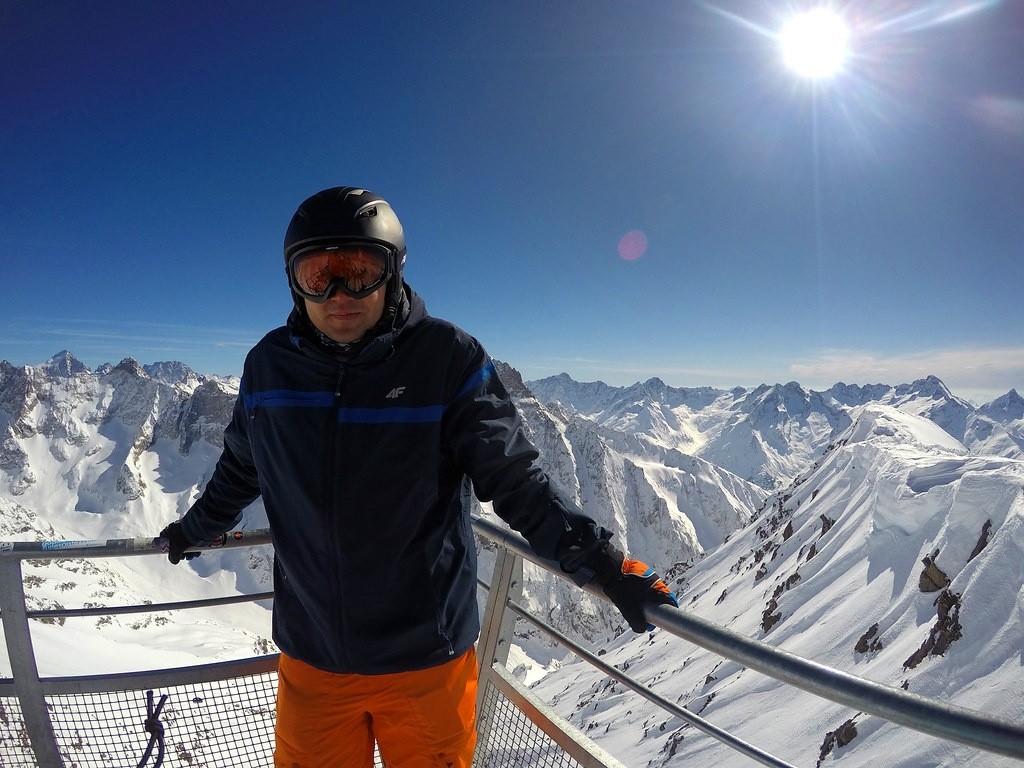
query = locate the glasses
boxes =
[288,239,393,304]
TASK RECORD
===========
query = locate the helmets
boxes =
[281,185,416,314]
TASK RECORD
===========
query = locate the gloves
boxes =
[159,519,204,565]
[592,542,679,634]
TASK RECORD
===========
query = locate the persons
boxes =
[158,186,682,768]
